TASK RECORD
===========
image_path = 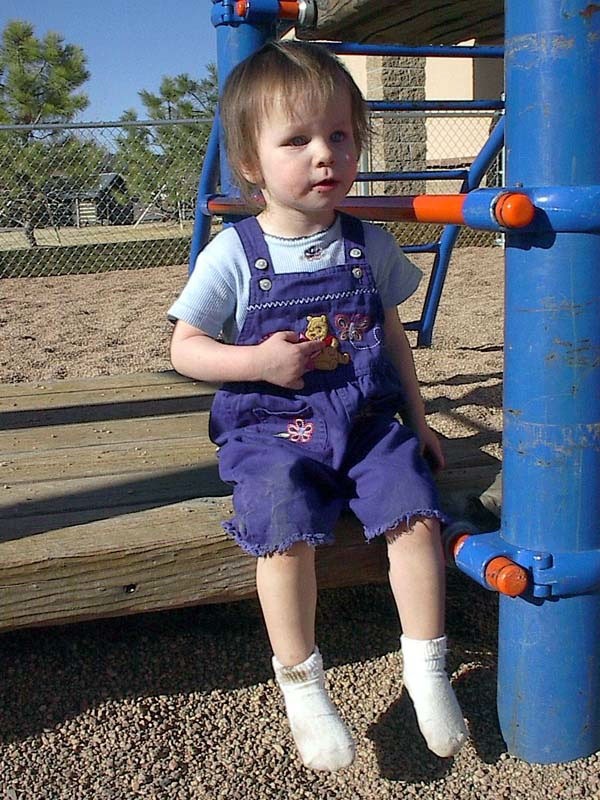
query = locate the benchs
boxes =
[0,372,502,632]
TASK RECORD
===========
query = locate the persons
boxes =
[169,39,470,776]
